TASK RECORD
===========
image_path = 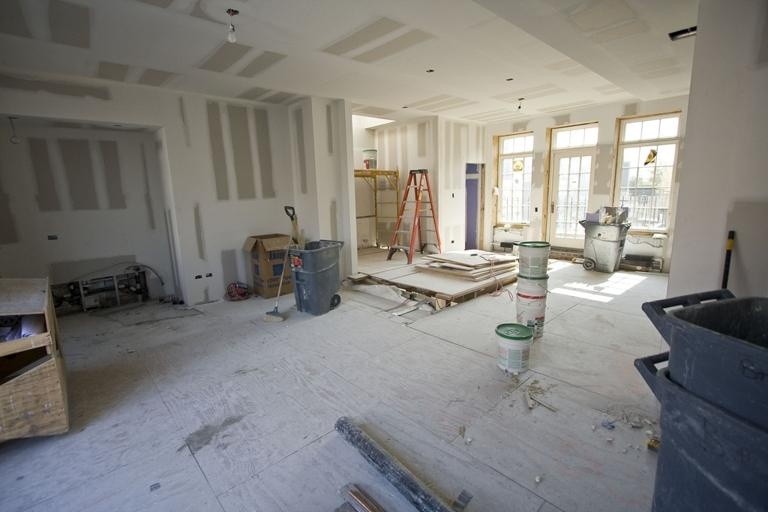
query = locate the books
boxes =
[242,233,298,298]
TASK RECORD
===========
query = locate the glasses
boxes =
[225,9,241,45]
[668,25,697,42]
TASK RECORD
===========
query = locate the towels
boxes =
[579,220,631,273]
[289,240,346,316]
[634,289,768,511]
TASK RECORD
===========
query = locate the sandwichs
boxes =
[264,216,302,323]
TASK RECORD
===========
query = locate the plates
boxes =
[48,255,147,317]
[0,276,69,442]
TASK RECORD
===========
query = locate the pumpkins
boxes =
[386,169,442,264]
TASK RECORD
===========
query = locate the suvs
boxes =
[362,149,377,170]
[516,240,550,338]
[494,323,534,373]
